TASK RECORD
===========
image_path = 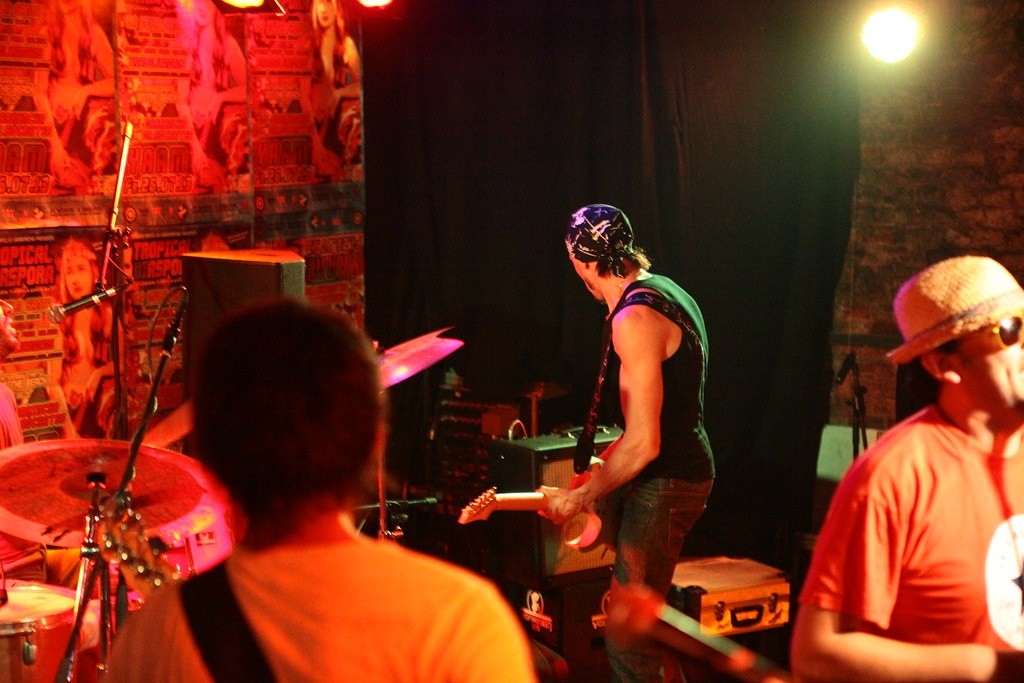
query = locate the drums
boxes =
[0,578,83,683]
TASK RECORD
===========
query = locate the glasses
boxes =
[967,315,1024,351]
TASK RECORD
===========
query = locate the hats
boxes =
[885,254,1024,366]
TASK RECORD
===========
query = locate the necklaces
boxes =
[618,274,624,294]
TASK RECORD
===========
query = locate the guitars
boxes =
[458,469,613,553]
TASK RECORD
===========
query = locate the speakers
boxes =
[180,249,305,403]
[489,426,629,592]
[502,577,613,683]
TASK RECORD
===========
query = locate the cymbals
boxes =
[0,438,224,549]
[377,326,463,389]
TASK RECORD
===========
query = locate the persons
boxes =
[790,256,1024,683]
[535,201,715,683]
[105,302,537,683]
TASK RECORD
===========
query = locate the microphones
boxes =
[46,282,129,324]
[836,352,856,385]
